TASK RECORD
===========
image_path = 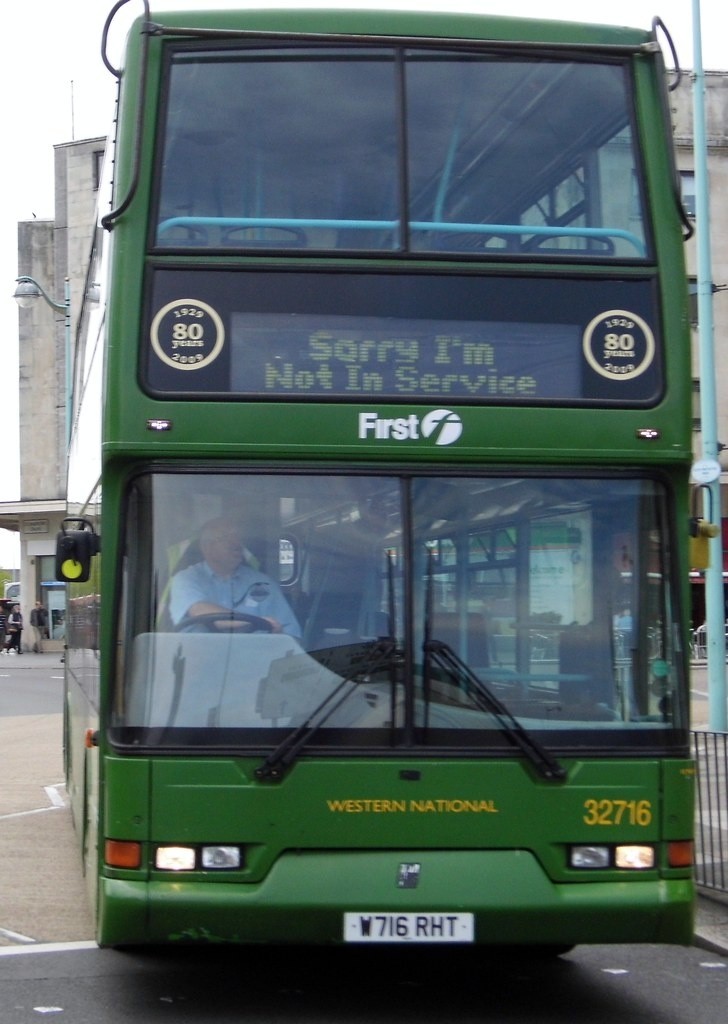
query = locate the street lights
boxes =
[15,271,73,460]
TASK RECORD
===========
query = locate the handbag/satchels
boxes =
[7,625,18,633]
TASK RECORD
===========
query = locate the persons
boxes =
[169,514,302,646]
[0,605,8,654]
[6,605,24,654]
[29,600,49,653]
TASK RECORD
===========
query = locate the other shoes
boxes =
[18,650,23,654]
[5,651,9,653]
[32,649,43,653]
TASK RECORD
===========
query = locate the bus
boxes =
[53,2,702,982]
[65,595,102,650]
